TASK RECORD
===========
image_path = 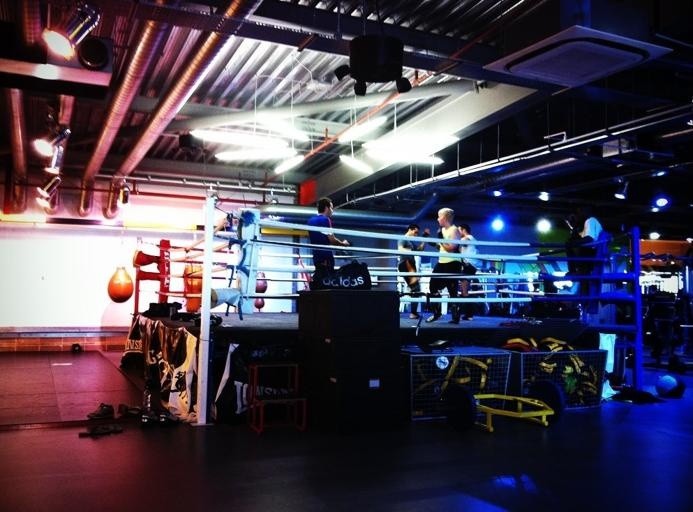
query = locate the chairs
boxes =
[244,356,309,434]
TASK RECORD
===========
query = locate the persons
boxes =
[530,278,548,293]
[397,222,430,319]
[567,204,603,273]
[458,225,478,319]
[425,207,463,325]
[642,285,659,357]
[305,196,349,290]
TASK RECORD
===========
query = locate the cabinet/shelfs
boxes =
[295,288,406,434]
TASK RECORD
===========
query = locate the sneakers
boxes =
[88,403,115,418]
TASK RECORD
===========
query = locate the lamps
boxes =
[29,96,70,197]
[41,3,99,58]
